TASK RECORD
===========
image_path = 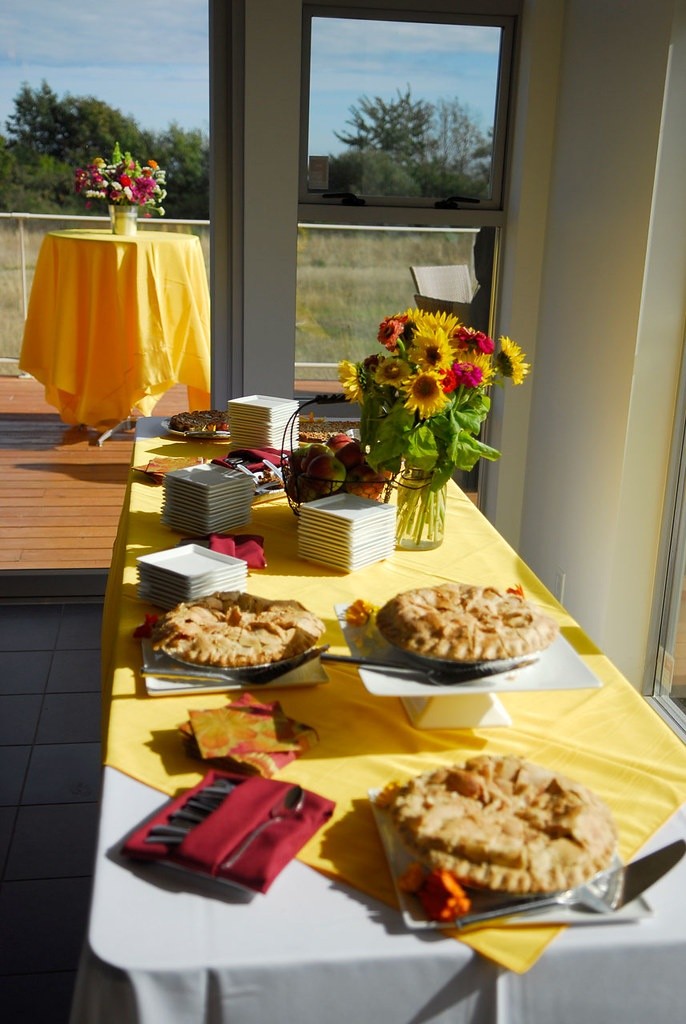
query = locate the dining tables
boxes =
[71,415,686,1023]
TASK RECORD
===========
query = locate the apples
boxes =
[287,443,346,499]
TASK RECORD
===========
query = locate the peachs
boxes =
[326,434,389,498]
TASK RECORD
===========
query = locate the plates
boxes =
[160,463,255,539]
[160,417,231,438]
[367,786,654,931]
[333,603,603,728]
[130,464,165,486]
[297,493,398,574]
[136,543,248,611]
[227,394,300,453]
[139,623,331,696]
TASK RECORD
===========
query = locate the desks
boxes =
[20,228,211,446]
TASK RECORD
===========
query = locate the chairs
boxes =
[410,262,475,329]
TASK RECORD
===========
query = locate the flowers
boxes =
[338,309,532,539]
[76,143,168,218]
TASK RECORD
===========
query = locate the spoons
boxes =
[218,784,303,870]
[455,840,686,929]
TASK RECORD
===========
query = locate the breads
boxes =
[169,409,230,431]
[374,754,618,893]
[299,420,362,443]
[377,582,559,662]
[153,591,324,667]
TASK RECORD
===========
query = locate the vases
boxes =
[108,203,138,234]
[393,466,447,552]
